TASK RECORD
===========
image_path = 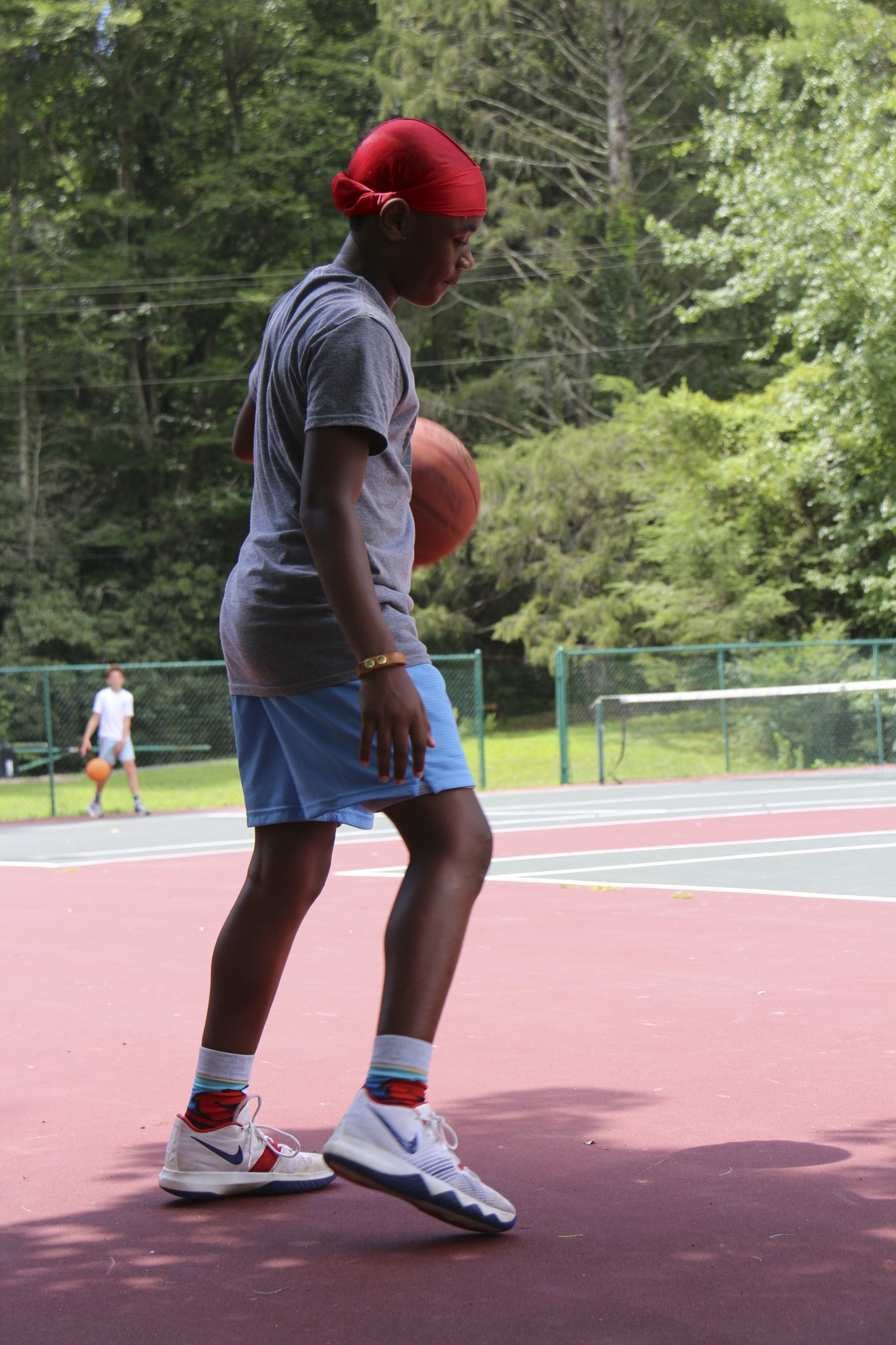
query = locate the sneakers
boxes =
[323,1088,518,1233]
[158,1093,338,1200]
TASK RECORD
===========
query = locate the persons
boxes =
[159,118,519,1233]
[80,664,151,818]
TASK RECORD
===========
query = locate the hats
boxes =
[331,118,487,217]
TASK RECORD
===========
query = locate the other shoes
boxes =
[135,806,149,817]
[89,803,103,818]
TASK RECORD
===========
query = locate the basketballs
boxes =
[86,757,110,781]
[409,417,483,568]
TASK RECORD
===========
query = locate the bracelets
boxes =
[354,651,407,676]
